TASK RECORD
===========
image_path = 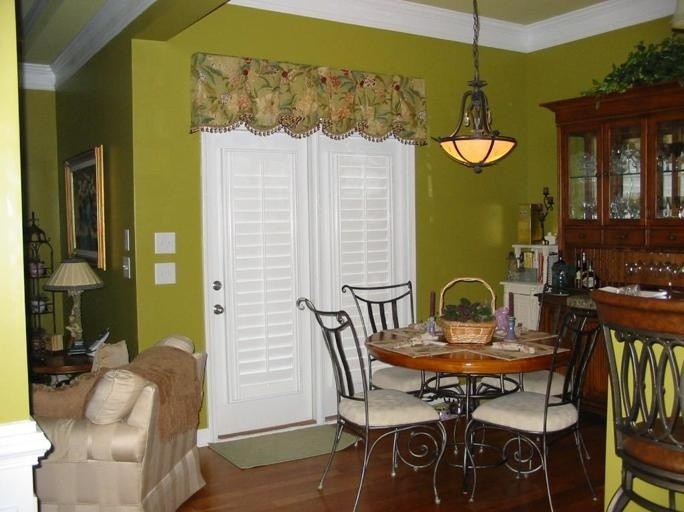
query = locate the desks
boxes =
[359,324,580,509]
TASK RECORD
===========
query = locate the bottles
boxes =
[551,256,569,296]
[573,251,597,291]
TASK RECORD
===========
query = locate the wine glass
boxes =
[577,140,684,220]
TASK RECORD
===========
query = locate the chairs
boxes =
[29,334,207,511]
[466,306,603,506]
[341,279,465,480]
[600,318,683,511]
[298,294,448,511]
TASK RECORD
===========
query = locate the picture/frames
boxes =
[64,144,105,273]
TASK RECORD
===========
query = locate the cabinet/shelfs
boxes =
[499,245,560,332]
[538,85,684,418]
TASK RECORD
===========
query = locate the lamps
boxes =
[43,258,103,355]
[431,0,517,174]
[537,186,555,245]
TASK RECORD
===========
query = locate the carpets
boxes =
[206,424,362,470]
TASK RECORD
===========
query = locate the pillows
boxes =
[29,339,129,416]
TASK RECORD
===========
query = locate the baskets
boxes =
[438,277,496,344]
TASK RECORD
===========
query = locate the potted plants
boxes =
[28,254,48,351]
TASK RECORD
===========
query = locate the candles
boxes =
[509,292,515,316]
[430,291,436,316]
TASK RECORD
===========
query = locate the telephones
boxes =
[86,328,110,355]
